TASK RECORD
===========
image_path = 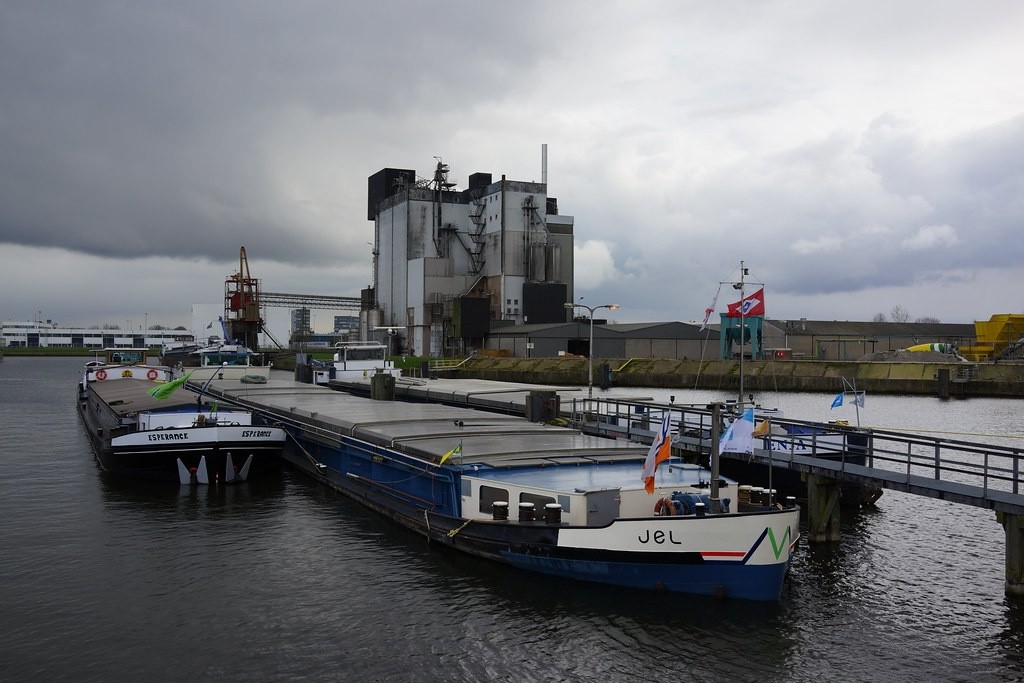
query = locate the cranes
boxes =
[218,247,286,351]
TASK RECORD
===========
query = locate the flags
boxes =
[641,408,671,496]
[830,392,844,410]
[726,288,765,318]
[439,443,462,466]
[751,418,770,439]
[700,287,720,331]
[145,371,193,401]
[849,391,865,408]
[709,409,754,464]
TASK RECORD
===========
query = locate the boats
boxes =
[158,326,802,626]
[589,260,870,476]
[78,347,287,484]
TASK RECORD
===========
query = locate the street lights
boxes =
[576,295,586,354]
[562,302,620,411]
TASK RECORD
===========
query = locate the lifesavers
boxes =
[96,369,107,380]
[654,497,676,516]
[146,369,159,380]
